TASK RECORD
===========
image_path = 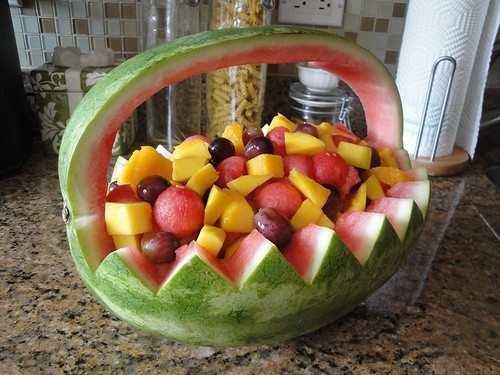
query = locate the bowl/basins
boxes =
[297,62,340,93]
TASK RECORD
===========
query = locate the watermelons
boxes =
[58,24,431,348]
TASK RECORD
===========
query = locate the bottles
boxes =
[287,82,352,130]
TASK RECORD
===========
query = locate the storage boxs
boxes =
[30,61,139,158]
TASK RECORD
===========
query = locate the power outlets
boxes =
[277,0,346,27]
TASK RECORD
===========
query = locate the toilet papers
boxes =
[394,0,500,160]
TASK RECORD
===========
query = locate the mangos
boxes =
[101,114,412,264]
